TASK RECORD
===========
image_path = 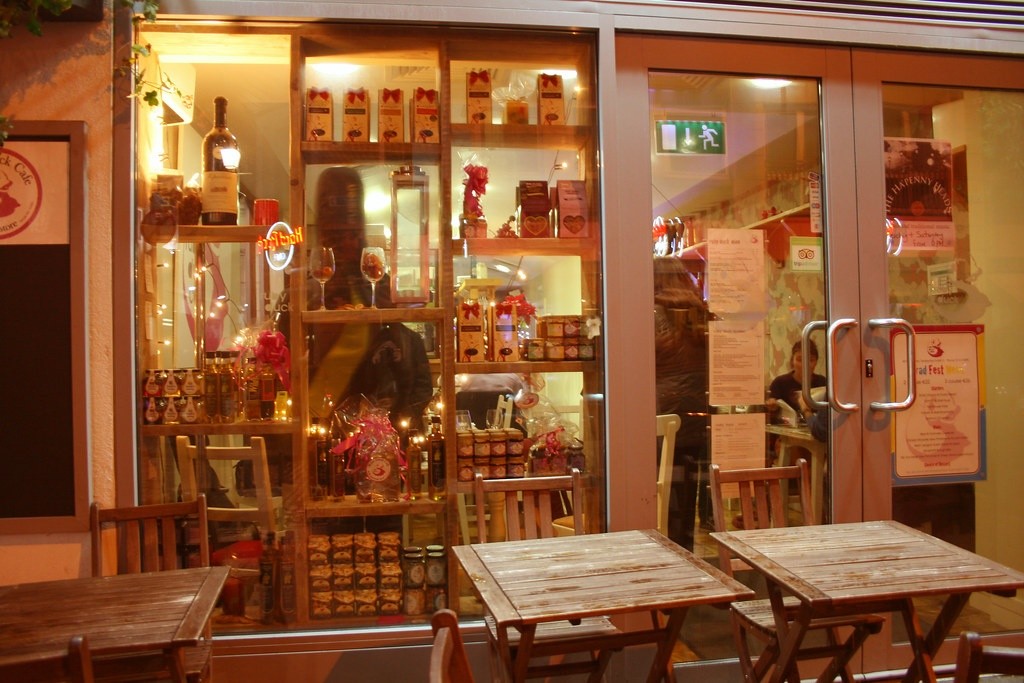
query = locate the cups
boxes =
[253,199,280,226]
[486,409,502,429]
[456,410,472,432]
[157,174,182,225]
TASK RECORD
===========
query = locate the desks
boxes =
[450,528,756,682]
[766,422,831,524]
[707,512,1023,683]
[0,566,233,683]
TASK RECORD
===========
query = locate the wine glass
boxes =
[361,247,386,311]
[310,248,336,311]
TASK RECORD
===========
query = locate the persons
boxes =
[797,395,828,442]
[652,258,713,551]
[350,321,432,436]
[768,338,826,458]
[456,373,528,436]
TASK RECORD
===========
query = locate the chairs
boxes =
[473,468,627,682]
[63,493,214,683]
[178,434,290,626]
[708,458,886,682]
[428,610,478,682]
[550,413,681,539]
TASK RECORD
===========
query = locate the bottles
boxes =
[331,418,356,501]
[205,351,222,424]
[246,358,290,421]
[260,531,295,623]
[428,417,446,501]
[269,438,293,496]
[202,96,239,225]
[309,416,327,502]
[407,429,422,495]
[222,352,245,422]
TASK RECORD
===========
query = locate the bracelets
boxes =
[801,408,810,415]
